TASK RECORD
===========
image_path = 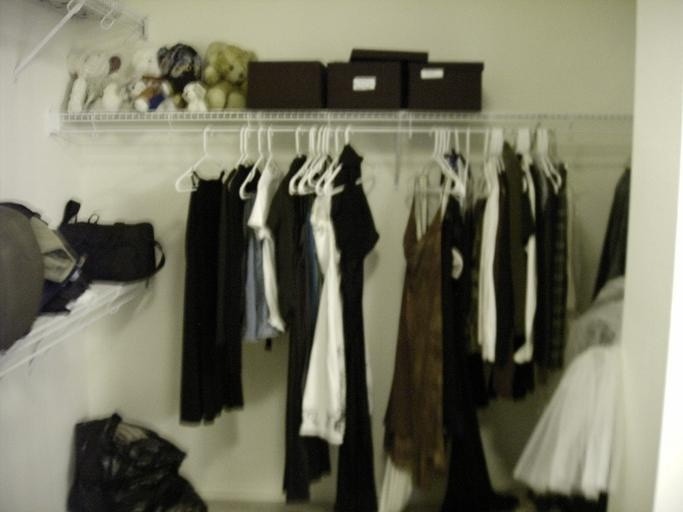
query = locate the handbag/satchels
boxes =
[57,199,165,281]
[66,413,209,511]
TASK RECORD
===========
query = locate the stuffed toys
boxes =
[59,38,256,113]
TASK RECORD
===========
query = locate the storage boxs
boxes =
[326,61,403,108]
[246,61,326,108]
[405,62,483,110]
